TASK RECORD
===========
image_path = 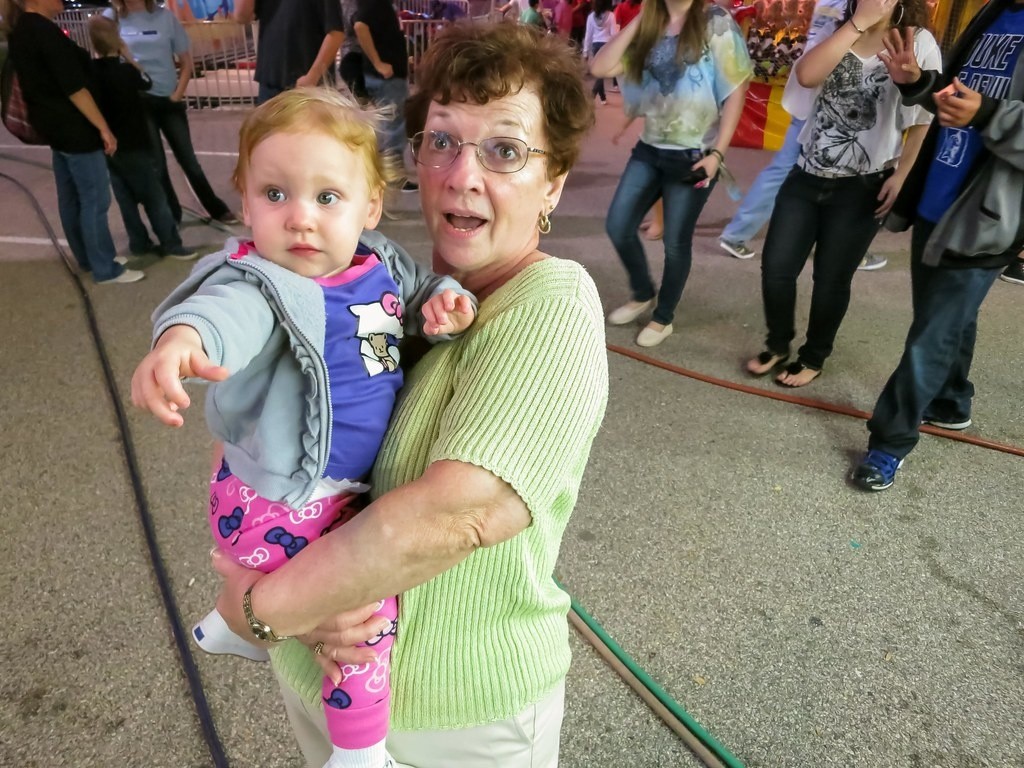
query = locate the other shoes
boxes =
[387,176,418,193]
[639,219,663,241]
[608,297,652,325]
[149,244,199,260]
[608,88,620,93]
[219,212,238,225]
[600,100,606,105]
[921,408,972,430]
[190,607,271,663]
[636,321,674,347]
[94,256,146,284]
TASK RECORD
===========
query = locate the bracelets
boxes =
[711,148,735,181]
[850,18,865,33]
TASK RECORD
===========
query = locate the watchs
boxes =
[242,586,296,643]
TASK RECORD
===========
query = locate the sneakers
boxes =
[720,239,755,259]
[856,250,888,270]
[1000,256,1024,286]
[851,447,904,490]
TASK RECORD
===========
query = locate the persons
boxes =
[5,0,420,285]
[716,1,1024,492]
[130,16,609,768]
[611,114,665,240]
[589,0,754,347]
[496,0,645,105]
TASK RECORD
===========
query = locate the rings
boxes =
[313,641,324,656]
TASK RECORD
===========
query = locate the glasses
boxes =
[405,129,553,174]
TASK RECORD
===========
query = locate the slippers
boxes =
[746,351,790,378]
[774,359,822,389]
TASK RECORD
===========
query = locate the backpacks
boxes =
[1,51,49,147]
